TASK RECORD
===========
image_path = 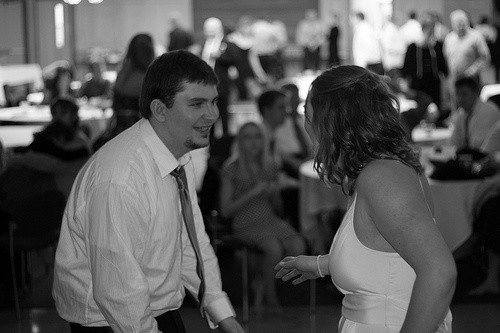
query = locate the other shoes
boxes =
[251,307,264,318]
[266,304,282,316]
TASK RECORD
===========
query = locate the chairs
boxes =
[216,233,317,323]
[3,82,34,107]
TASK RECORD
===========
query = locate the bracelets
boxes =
[316,254,326,278]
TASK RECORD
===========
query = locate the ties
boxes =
[169,166,205,319]
[291,115,309,155]
[463,111,472,147]
[269,139,276,170]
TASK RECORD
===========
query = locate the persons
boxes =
[0,6,500,310]
[272,65,459,333]
[52,48,247,332]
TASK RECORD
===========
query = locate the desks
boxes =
[0,104,114,147]
[298,145,500,314]
